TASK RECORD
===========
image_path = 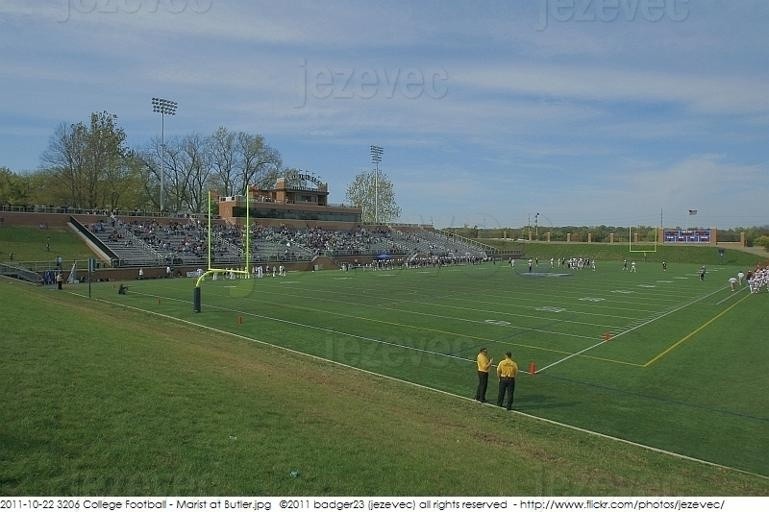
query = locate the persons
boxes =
[39,222,48,230]
[697,265,706,280]
[118,284,127,295]
[728,265,769,294]
[662,261,667,271]
[56,256,63,266]
[508,255,515,266]
[474,347,518,410]
[551,257,596,272]
[622,259,636,273]
[528,257,538,271]
[56,272,64,290]
[91,207,495,279]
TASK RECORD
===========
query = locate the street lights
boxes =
[370,143,384,223]
[151,97,178,211]
